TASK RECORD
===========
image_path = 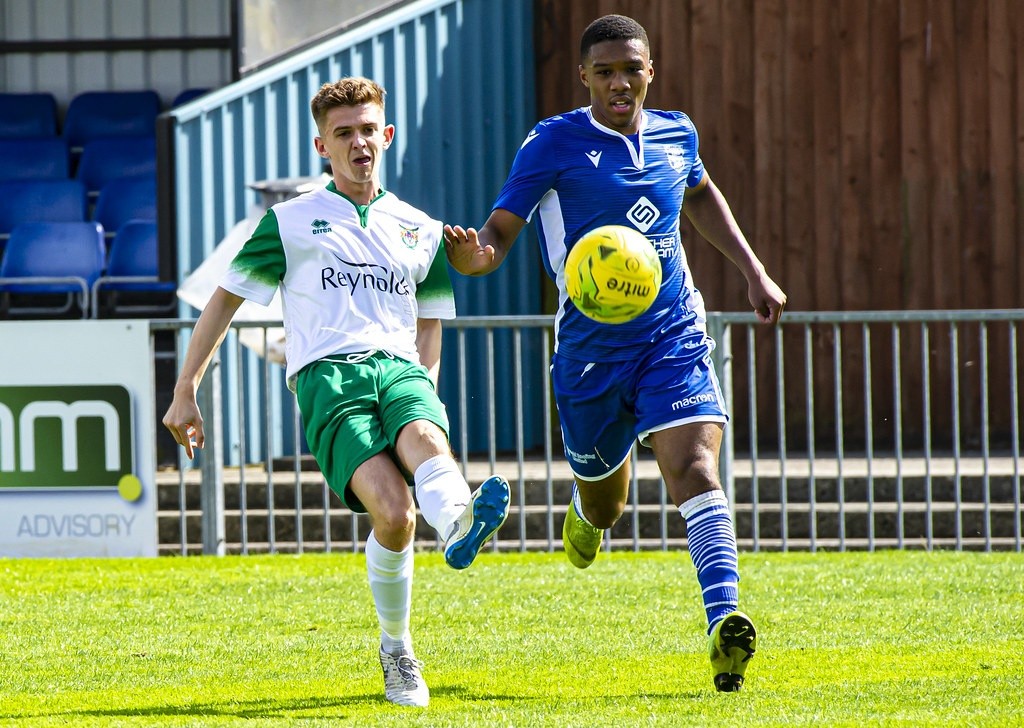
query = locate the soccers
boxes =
[564,223,662,325]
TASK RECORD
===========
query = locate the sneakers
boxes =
[561,481,603,569]
[441,475,512,570]
[707,611,760,694]
[377,643,432,708]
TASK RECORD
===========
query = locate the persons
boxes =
[440,15,788,691]
[161,76,511,709]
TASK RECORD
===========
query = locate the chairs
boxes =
[0,87,217,319]
